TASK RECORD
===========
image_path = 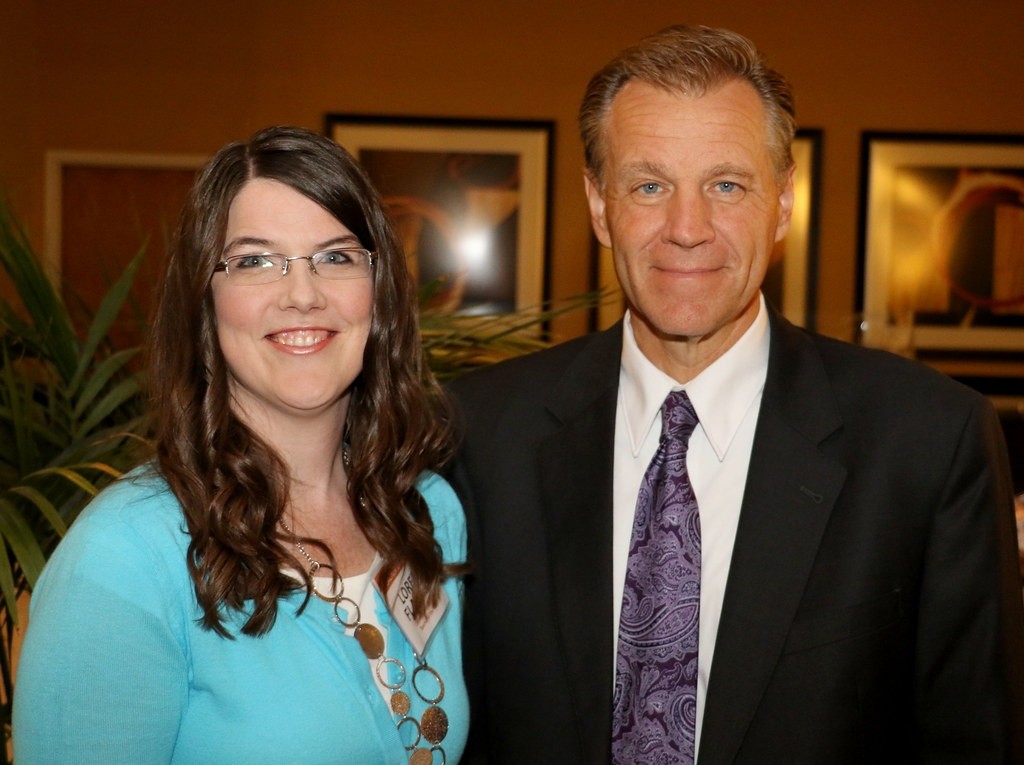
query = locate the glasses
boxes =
[213,248,378,286]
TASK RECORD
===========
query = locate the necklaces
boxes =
[279,456,449,765]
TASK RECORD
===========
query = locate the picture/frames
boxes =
[586,125,826,337]
[852,125,1023,363]
[322,109,557,373]
[45,146,218,309]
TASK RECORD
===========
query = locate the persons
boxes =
[12,126,469,765]
[442,24,1024,765]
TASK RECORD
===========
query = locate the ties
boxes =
[611,390,703,765]
